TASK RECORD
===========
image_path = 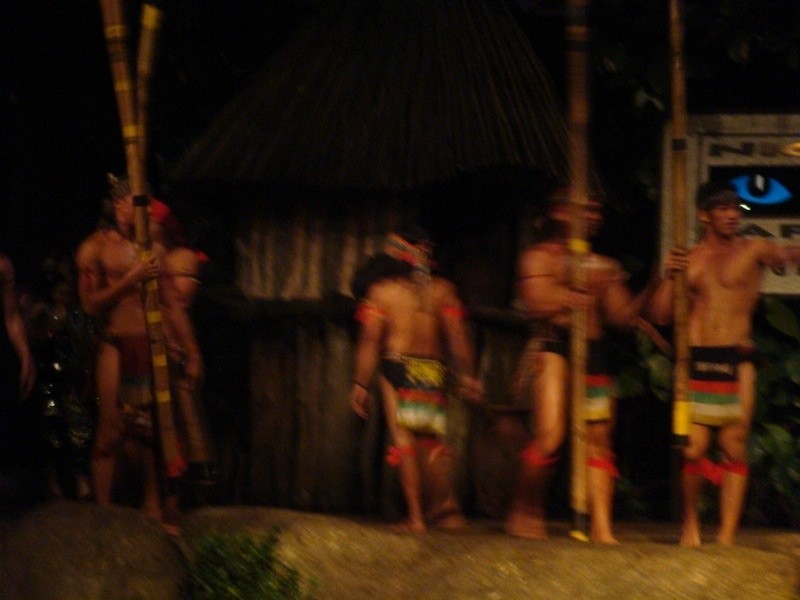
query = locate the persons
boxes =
[0,175,214,515]
[503,186,662,536]
[350,223,483,534]
[666,178,800,549]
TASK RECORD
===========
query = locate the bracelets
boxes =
[353,378,369,392]
